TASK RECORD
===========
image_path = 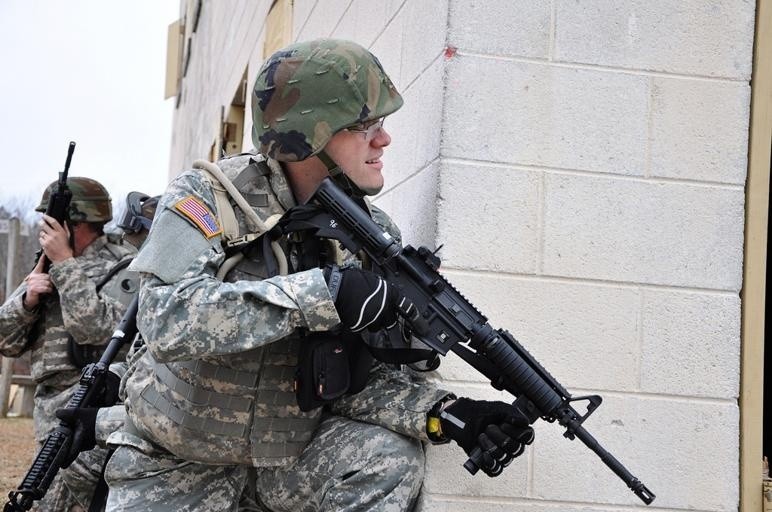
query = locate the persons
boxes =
[0,178,140,511]
[104,38,535,512]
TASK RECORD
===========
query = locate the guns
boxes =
[3,295,138,511]
[17,142,75,352]
[260,178,656,506]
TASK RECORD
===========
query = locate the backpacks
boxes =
[120,169,243,248]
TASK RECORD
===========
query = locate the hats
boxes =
[35,177,112,222]
[251,38,403,162]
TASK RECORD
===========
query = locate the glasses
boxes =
[343,117,385,142]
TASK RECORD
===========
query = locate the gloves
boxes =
[440,397,535,477]
[324,263,429,336]
